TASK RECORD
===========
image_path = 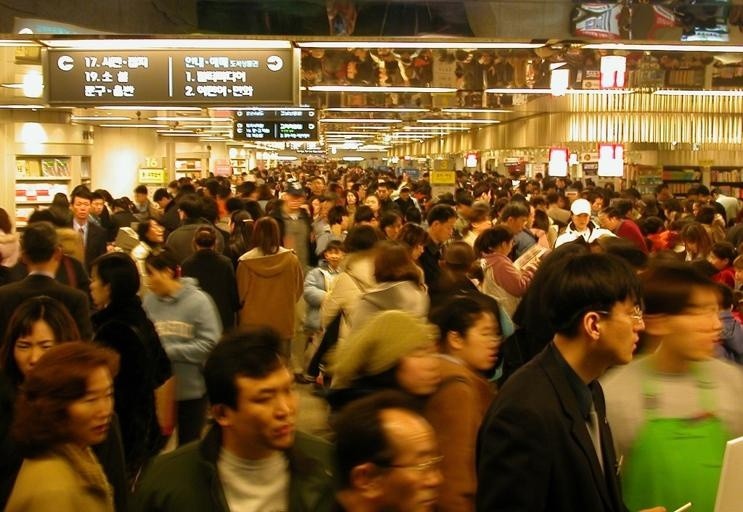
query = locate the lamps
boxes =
[595,142,625,179]
[546,146,571,179]
[464,152,481,168]
[599,53,628,91]
[549,65,571,99]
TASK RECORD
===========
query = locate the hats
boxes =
[115,227,139,250]
[328,310,437,391]
[400,186,408,191]
[287,181,304,196]
[571,198,593,217]
[520,218,528,226]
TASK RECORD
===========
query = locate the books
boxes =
[712,168,743,182]
[663,168,701,181]
[719,185,743,198]
[668,182,691,194]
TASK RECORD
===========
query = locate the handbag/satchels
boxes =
[480,258,522,319]
[295,267,330,334]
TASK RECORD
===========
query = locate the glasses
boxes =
[381,454,445,472]
[468,333,502,344]
[597,308,642,322]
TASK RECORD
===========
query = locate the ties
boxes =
[79,229,85,246]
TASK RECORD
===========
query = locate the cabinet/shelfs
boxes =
[228,146,250,178]
[1,143,93,234]
[136,156,176,203]
[660,163,743,209]
[175,151,211,184]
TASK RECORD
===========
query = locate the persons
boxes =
[532,197,546,214]
[586,192,604,218]
[455,193,472,232]
[476,253,669,512]
[678,222,711,263]
[644,204,659,222]
[734,255,742,292]
[716,284,742,362]
[601,264,742,511]
[551,195,571,226]
[529,187,541,203]
[711,241,736,287]
[605,183,613,191]
[493,200,506,225]
[510,235,639,334]
[697,186,728,228]
[475,226,542,328]
[302,47,505,87]
[696,207,725,243]
[424,205,461,296]
[586,182,594,190]
[481,174,488,182]
[513,203,538,259]
[554,198,615,248]
[425,293,501,511]
[663,199,681,227]
[611,199,634,220]
[462,204,491,250]
[692,200,705,216]
[711,188,738,227]
[0,164,440,511]
[502,178,512,198]
[437,241,499,314]
[437,193,455,209]
[655,184,673,220]
[599,207,649,254]
[456,170,466,189]
[545,183,557,196]
[473,181,492,206]
[532,173,544,189]
[500,200,529,262]
[514,176,528,196]
[533,209,556,248]
[643,218,661,240]
